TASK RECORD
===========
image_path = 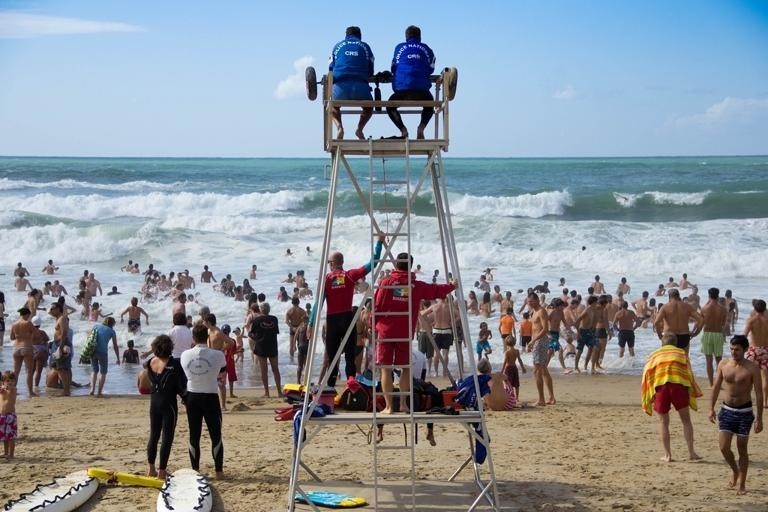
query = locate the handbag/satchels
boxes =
[80,328,98,363]
[339,376,438,411]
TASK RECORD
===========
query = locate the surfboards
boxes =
[296,490,367,508]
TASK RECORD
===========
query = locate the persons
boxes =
[147,326,232,480]
[632,273,768,495]
[0,370,18,458]
[305,237,469,446]
[329,26,374,139]
[466,270,640,411]
[386,25,435,139]
[0,246,313,410]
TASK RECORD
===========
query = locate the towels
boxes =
[640,346,703,416]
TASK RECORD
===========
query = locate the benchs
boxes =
[324,66,450,153]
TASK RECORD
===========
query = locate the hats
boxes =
[31,315,41,326]
